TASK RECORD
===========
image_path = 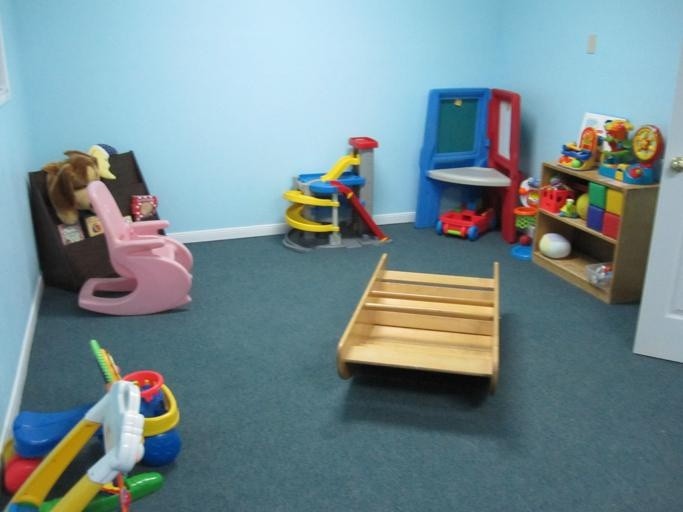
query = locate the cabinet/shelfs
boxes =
[23,153,167,288]
[532,161,659,304]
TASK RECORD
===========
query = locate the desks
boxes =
[414,87,527,243]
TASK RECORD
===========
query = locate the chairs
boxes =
[78,180,196,315]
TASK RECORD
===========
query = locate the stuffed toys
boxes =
[40,147,104,224]
